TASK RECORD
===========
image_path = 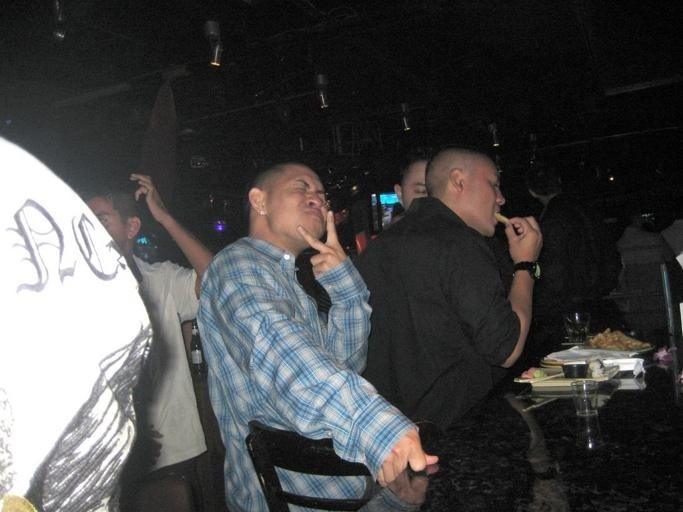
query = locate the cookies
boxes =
[495,212,509,225]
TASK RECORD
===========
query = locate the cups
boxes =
[575,415,606,450]
[571,380,600,418]
[564,312,590,344]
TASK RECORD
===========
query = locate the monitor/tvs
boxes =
[368,190,406,236]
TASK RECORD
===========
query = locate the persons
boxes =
[388,149,431,227]
[349,141,545,447]
[608,198,682,336]
[195,158,437,512]
[0,136,216,512]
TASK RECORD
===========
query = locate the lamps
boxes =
[50,1,70,42]
[488,120,501,148]
[202,20,224,66]
[398,102,413,132]
[313,72,330,110]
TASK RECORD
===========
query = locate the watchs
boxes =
[511,261,541,280]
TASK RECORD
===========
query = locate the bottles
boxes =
[187,320,207,374]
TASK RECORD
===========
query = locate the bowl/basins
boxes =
[562,362,588,379]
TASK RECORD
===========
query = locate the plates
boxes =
[514,344,655,387]
[514,386,620,400]
